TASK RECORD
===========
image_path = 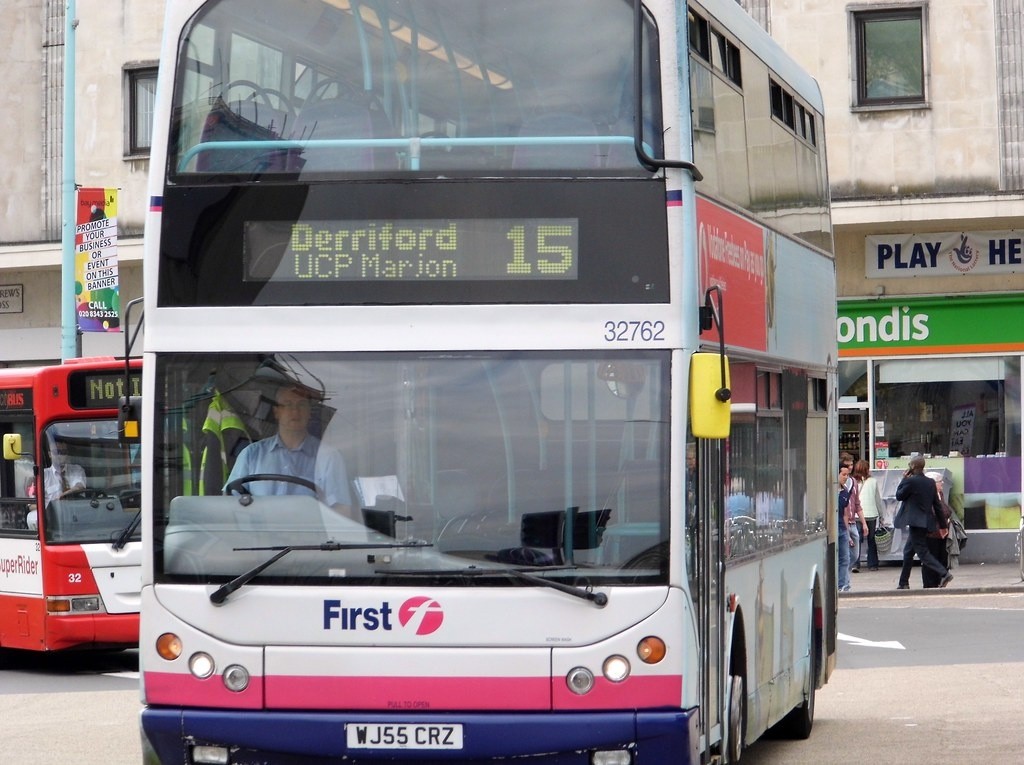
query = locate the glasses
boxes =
[933,479,943,483]
[908,463,914,466]
[274,400,308,409]
[50,449,68,456]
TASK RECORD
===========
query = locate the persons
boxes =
[686,445,785,531]
[893,456,953,589]
[851,460,885,573]
[27,440,86,530]
[221,384,351,519]
[838,452,869,591]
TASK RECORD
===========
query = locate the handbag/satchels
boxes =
[927,519,948,539]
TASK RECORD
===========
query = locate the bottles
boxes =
[839,433,860,450]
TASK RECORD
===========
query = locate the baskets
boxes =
[875,523,892,551]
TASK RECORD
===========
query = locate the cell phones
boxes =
[905,467,912,475]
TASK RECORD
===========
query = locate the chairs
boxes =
[220,76,633,173]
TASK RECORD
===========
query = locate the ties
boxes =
[56,471,70,492]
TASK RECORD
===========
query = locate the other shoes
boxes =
[870,567,878,571]
[852,567,860,573]
[897,584,909,589]
[838,585,850,594]
[938,573,953,588]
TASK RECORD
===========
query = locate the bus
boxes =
[0,355,201,654]
[121,0,842,765]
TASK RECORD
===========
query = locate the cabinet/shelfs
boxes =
[837,430,868,465]
